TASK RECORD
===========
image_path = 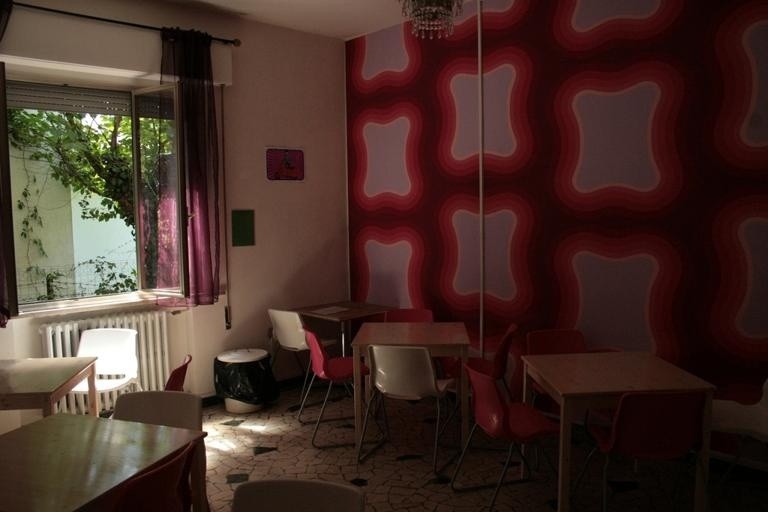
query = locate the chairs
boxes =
[66,328,145,395]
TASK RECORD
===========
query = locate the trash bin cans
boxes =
[217,345,269,414]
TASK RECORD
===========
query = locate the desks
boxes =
[1,356,209,512]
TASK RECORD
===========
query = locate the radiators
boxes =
[36,308,171,417]
